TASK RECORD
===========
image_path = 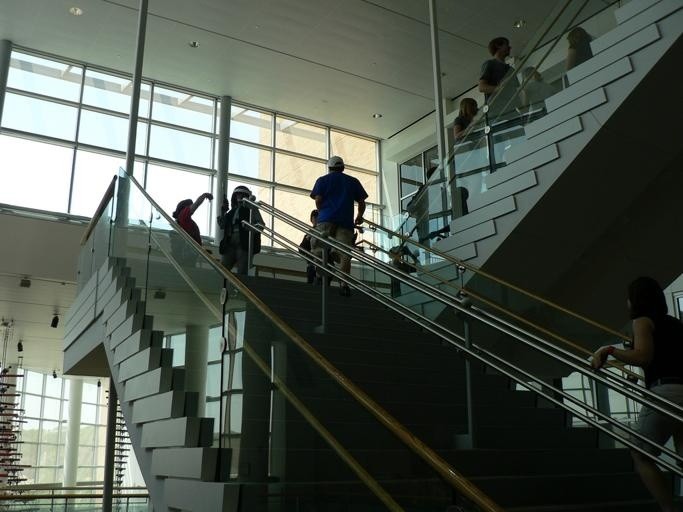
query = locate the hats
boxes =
[327,156,344,167]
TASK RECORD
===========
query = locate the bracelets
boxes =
[608,345,616,355]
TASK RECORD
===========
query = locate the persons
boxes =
[218,186,264,276]
[299,156,369,296]
[593,274,683,512]
[172,192,213,267]
